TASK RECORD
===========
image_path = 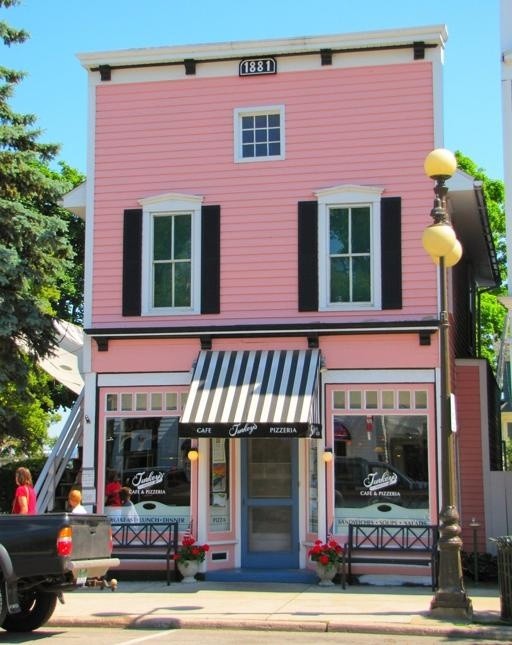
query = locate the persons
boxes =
[106,469,121,508]
[121,487,139,525]
[12,467,36,514]
[69,490,86,514]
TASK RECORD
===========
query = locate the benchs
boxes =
[341,523,440,593]
[108,521,179,586]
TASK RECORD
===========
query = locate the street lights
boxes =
[424,147,473,609]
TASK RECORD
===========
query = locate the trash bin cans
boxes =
[488,534,511,624]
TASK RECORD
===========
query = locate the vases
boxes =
[316,561,337,585]
[177,559,201,583]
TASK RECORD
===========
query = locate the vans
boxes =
[120,467,187,505]
[335,457,429,508]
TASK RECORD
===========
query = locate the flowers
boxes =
[306,540,344,576]
[171,536,209,569]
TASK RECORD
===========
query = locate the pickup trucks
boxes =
[0,515,121,635]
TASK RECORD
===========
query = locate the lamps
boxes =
[188,447,198,460]
[322,448,332,462]
